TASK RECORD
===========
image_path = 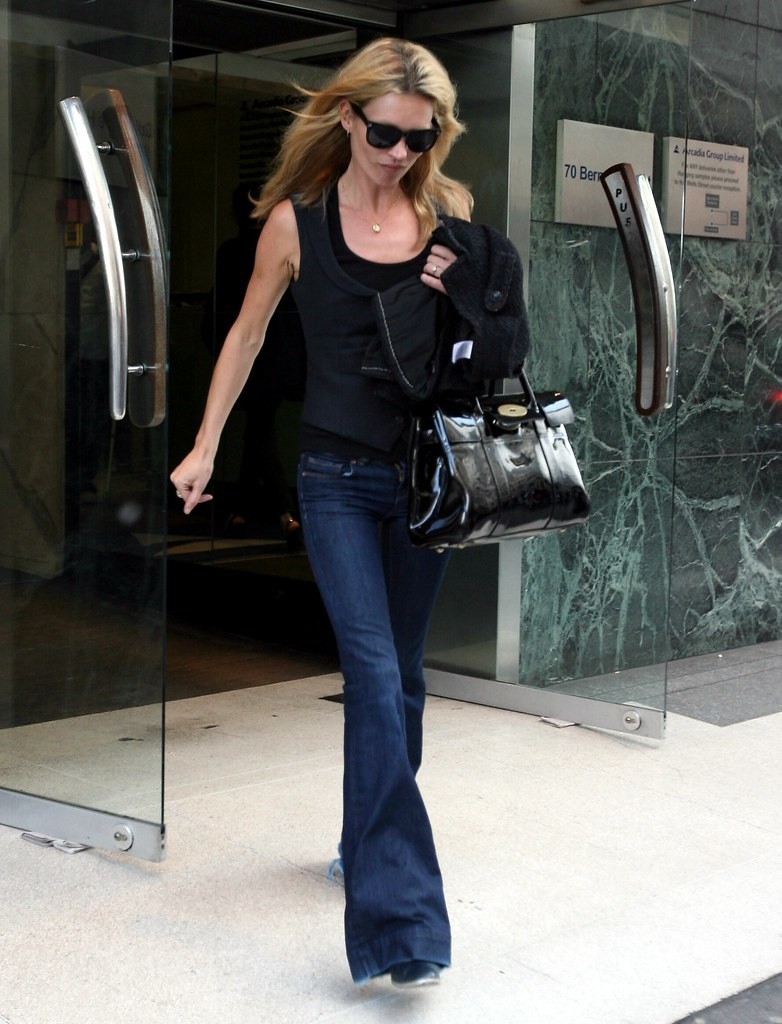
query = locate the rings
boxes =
[431,265,439,274]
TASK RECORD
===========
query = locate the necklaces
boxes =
[340,176,402,233]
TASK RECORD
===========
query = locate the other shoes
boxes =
[391,960,441,986]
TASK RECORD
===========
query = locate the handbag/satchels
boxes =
[406,322,589,554]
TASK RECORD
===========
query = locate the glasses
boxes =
[350,99,442,153]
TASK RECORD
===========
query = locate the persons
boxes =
[168,40,532,994]
